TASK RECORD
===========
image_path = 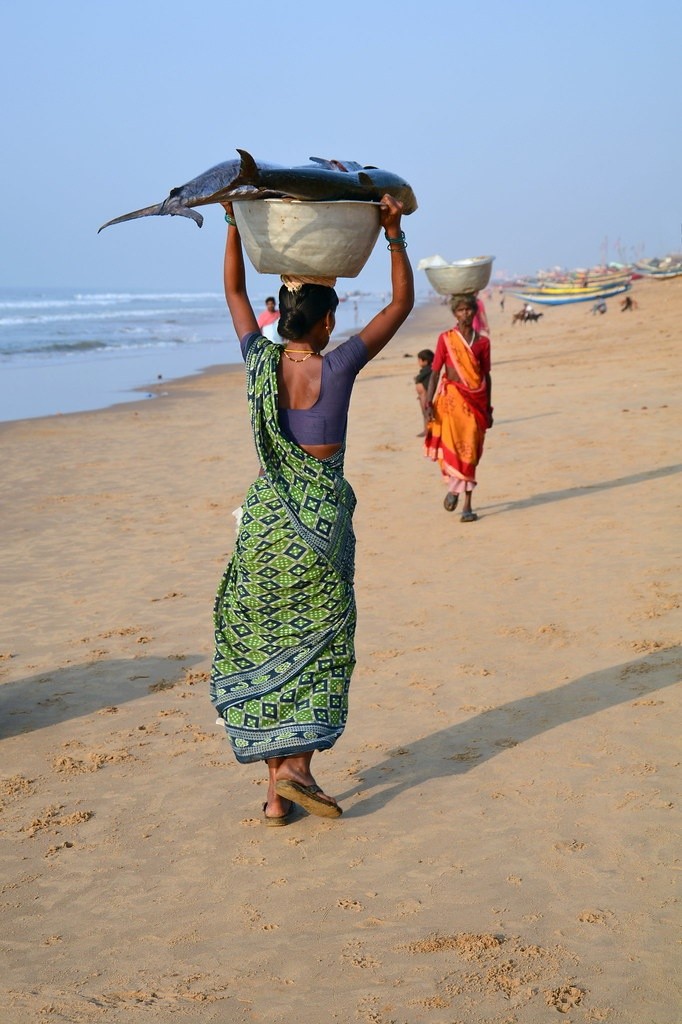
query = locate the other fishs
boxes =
[94,150,419,233]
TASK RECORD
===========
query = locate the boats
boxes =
[495,252,682,306]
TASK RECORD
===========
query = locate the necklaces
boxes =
[281,349,321,364]
[456,326,473,341]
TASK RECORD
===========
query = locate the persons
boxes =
[592,296,610,318]
[252,297,288,362]
[413,288,494,529]
[495,288,513,310]
[513,299,545,325]
[207,194,416,828]
[617,296,637,315]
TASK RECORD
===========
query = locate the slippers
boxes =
[444,492,458,511]
[274,779,342,819]
[262,799,296,826]
[460,513,476,522]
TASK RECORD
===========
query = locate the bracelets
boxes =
[423,399,435,410]
[490,405,495,413]
[384,227,409,251]
[222,211,239,227]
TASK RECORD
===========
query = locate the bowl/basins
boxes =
[425,255,496,295]
[232,197,388,279]
